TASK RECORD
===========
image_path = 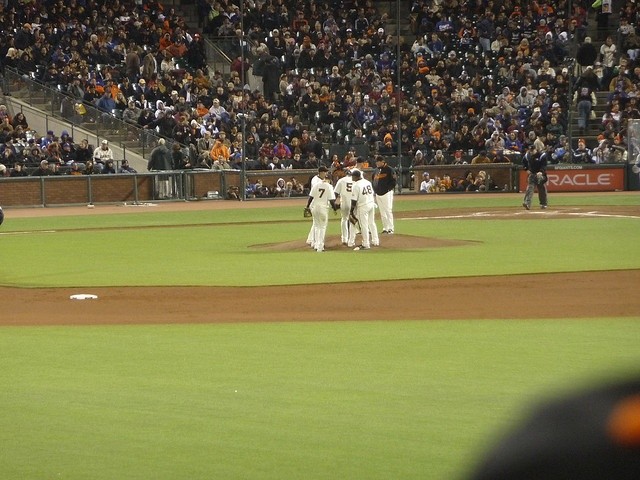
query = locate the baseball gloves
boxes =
[349,216,359,225]
[533,172,547,184]
[303,208,312,218]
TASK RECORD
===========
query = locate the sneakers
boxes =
[382,230,386,233]
[388,229,393,233]
[523,203,530,211]
[540,205,548,209]
[353,245,366,250]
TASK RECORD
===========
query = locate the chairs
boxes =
[96,64,105,73]
[109,108,122,118]
[36,64,44,72]
[409,171,414,190]
[280,93,332,137]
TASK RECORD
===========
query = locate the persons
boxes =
[523,145,549,209]
[0,1,342,171]
[0,105,137,177]
[335,0,523,163]
[440,171,501,193]
[350,170,379,249]
[306,167,329,244]
[524,4,640,145]
[304,174,337,253]
[254,177,304,197]
[333,169,355,247]
[349,157,369,179]
[420,171,434,194]
[547,131,628,164]
[523,128,547,152]
[372,156,396,234]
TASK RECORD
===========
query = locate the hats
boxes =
[432,89,437,92]
[494,132,499,134]
[172,91,178,94]
[596,135,603,139]
[273,29,279,33]
[538,88,546,93]
[255,118,261,123]
[219,131,225,134]
[350,170,361,176]
[577,139,585,143]
[415,150,422,154]
[318,166,329,172]
[534,107,540,112]
[193,34,200,38]
[497,150,503,155]
[422,172,429,176]
[128,100,135,103]
[356,156,365,162]
[480,150,486,155]
[158,138,165,144]
[104,86,111,93]
[213,98,219,104]
[552,103,560,108]
[47,130,53,135]
[378,28,384,32]
[272,104,277,110]
[545,32,553,38]
[384,133,392,139]
[503,86,510,91]
[614,136,620,140]
[436,150,442,153]
[247,135,253,140]
[528,145,536,150]
[204,131,212,135]
[310,133,316,137]
[101,140,108,144]
[139,79,145,84]
[61,130,68,135]
[41,160,48,164]
[345,28,353,33]
[560,31,568,39]
[244,83,250,89]
[450,50,455,56]
[469,109,474,113]
[170,75,176,80]
[235,153,241,159]
[364,95,368,99]
[227,83,234,88]
[333,65,338,70]
[175,38,181,44]
[562,68,568,73]
[456,152,462,157]
[374,156,384,162]
